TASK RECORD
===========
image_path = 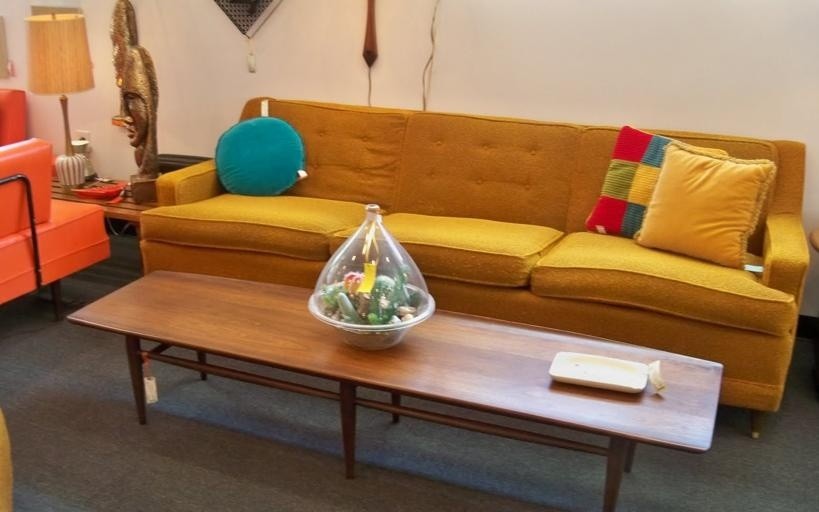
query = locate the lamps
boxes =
[23,12,99,196]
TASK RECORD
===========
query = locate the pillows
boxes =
[581,122,730,242]
[210,116,308,197]
[631,139,776,271]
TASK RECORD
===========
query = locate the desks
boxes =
[47,175,162,276]
[65,264,730,512]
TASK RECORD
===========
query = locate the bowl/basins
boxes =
[308,282,435,352]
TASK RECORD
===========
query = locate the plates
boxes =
[70,186,121,200]
[548,352,648,393]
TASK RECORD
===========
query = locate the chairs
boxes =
[0,88,29,145]
[0,137,115,326]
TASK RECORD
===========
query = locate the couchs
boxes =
[136,91,814,446]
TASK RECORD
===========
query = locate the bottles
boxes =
[73,140,97,180]
